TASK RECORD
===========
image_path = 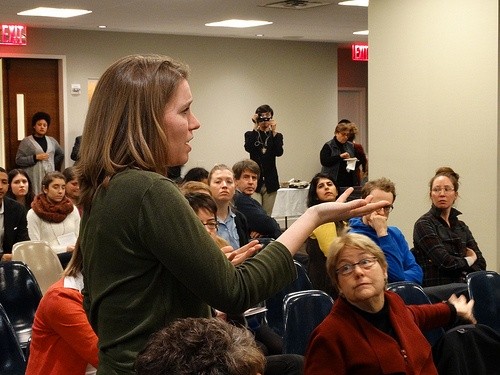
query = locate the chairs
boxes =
[466,271,500,331]
[282,290,336,356]
[0,304,27,375]
[13,240,64,297]
[263,260,314,337]
[434,325,500,375]
[387,282,445,341]
[0,261,43,357]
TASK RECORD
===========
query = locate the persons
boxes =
[303,173,352,286]
[70,53,395,375]
[244,104,284,217]
[412,167,487,286]
[132,317,266,375]
[0,110,84,262]
[320,119,368,188]
[302,234,479,375]
[348,177,424,288]
[23,268,100,375]
[165,160,282,356]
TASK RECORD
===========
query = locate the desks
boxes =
[252,182,310,230]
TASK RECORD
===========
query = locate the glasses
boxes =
[375,204,393,213]
[341,132,350,136]
[335,256,377,274]
[200,219,220,229]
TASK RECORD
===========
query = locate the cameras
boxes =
[255,112,270,122]
